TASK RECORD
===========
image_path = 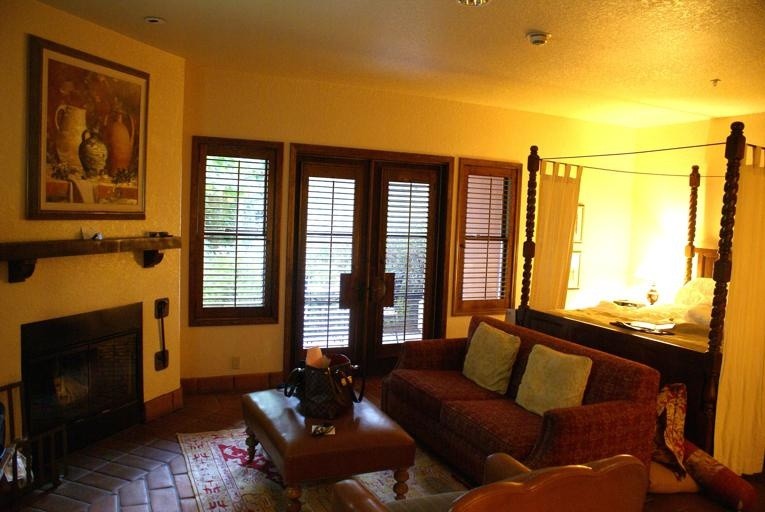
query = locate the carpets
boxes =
[177,427,468,512]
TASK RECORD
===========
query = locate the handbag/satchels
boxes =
[284,354,364,418]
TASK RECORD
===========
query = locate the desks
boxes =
[45,163,137,204]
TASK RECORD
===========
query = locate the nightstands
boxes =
[613,299,645,307]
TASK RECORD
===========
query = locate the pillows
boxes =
[677,277,716,304]
[462,322,521,396]
[515,343,593,417]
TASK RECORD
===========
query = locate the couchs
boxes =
[380,315,661,488]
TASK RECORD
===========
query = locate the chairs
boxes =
[332,452,647,512]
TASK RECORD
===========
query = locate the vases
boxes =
[53,103,135,176]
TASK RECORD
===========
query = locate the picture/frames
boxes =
[572,204,585,244]
[567,250,583,290]
[28,33,150,219]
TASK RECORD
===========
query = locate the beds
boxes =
[515,120,765,476]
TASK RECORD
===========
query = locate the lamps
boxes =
[647,281,659,304]
